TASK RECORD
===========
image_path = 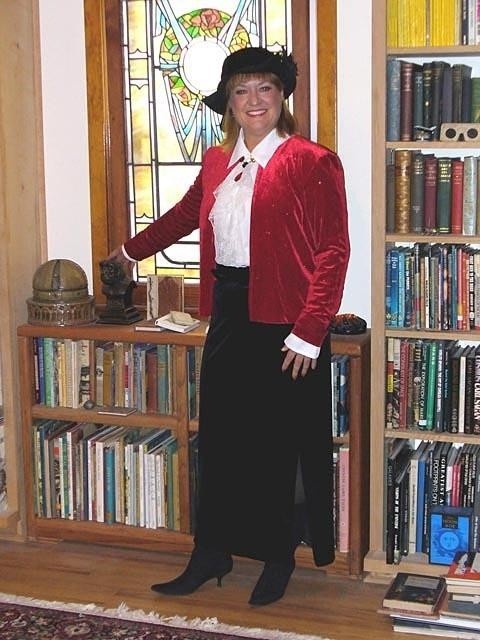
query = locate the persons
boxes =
[104,49,349,606]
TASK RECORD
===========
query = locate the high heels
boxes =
[151,544,233,595]
[248,542,296,606]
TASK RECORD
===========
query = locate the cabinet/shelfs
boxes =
[16,322,370,580]
[1,0,48,539]
[363,0,480,588]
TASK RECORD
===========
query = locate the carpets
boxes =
[0,592,328,640]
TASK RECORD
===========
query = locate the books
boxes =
[330,357,348,555]
[135,275,200,335]
[385,148,476,331]
[385,337,477,563]
[32,338,202,535]
[386,3,477,142]
[385,550,478,635]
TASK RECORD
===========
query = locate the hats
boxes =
[200,48,295,116]
